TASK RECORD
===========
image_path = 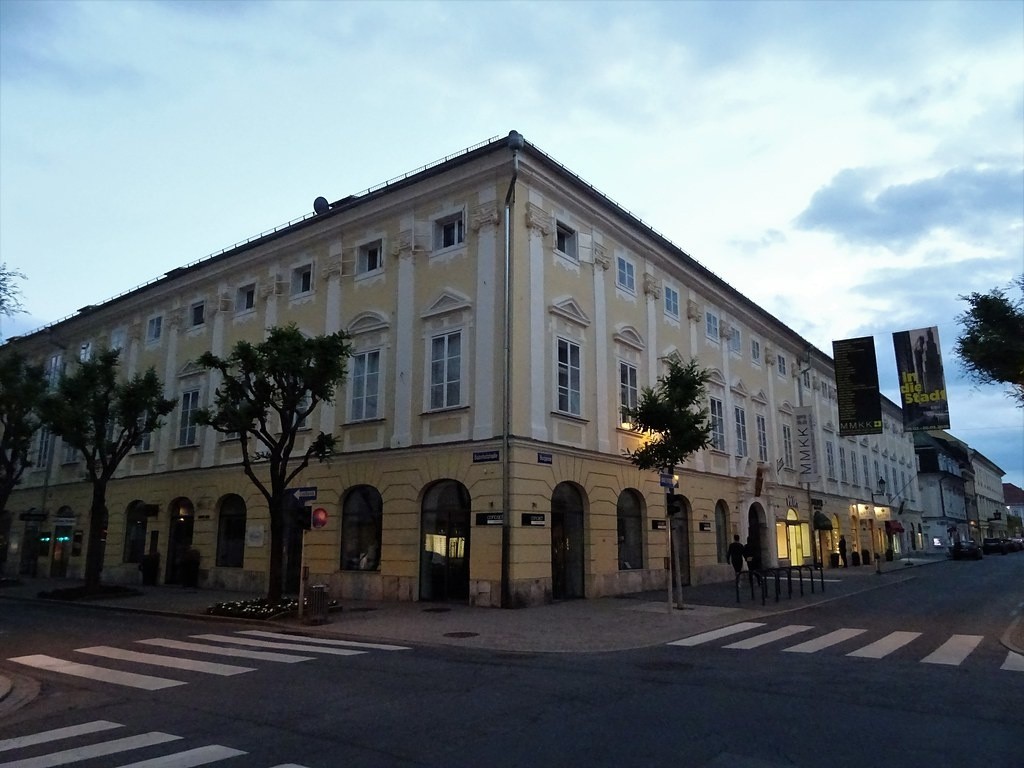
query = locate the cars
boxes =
[982,536,1023,555]
[952,540,984,561]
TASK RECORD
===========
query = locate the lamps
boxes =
[988,510,1001,522]
[871,476,886,503]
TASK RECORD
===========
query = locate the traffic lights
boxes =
[304,500,329,531]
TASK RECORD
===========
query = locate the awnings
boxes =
[813,511,833,532]
[885,520,905,533]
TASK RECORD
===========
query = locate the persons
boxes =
[839,535,848,568]
[340,536,379,572]
[726,535,764,588]
